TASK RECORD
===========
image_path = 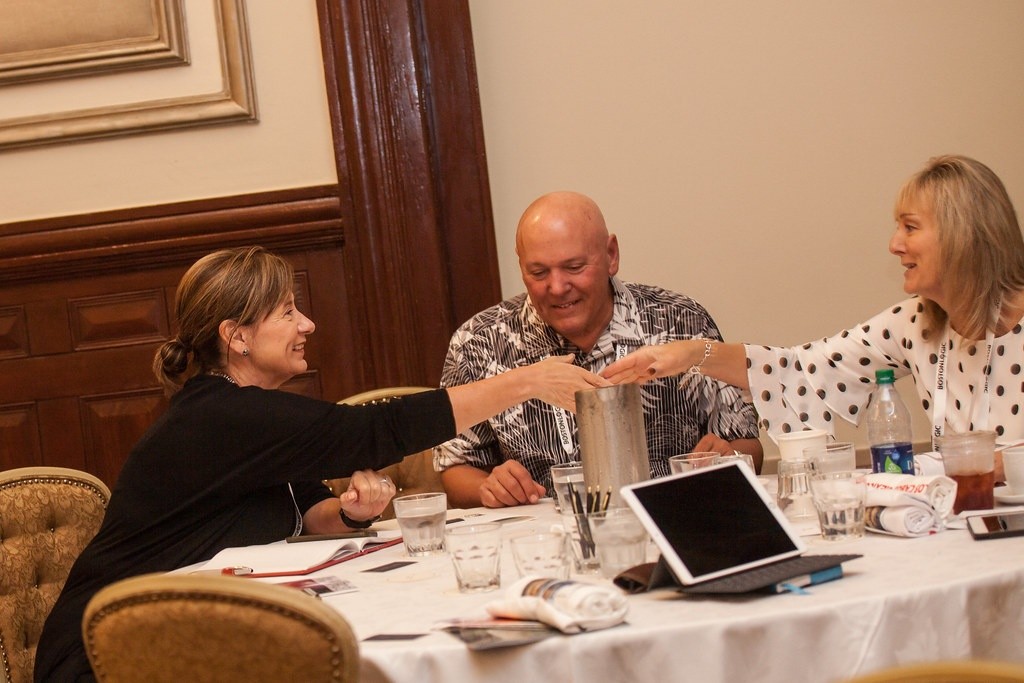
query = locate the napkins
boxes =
[481,573,627,634]
[866,471,959,539]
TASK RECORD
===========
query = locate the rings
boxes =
[380,479,390,485]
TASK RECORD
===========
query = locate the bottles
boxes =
[866,370,915,477]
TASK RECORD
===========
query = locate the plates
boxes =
[993,487,1024,504]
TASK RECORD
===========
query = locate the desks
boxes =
[149,462,1024,683]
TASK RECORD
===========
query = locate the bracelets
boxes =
[340,508,381,528]
[689,341,711,374]
[734,450,742,454]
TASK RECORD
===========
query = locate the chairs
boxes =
[322,386,452,517]
[0,465,114,683]
[85,572,362,683]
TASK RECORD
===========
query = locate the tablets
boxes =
[618,459,807,589]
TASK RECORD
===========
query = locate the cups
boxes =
[937,431,997,515]
[776,430,827,461]
[777,460,823,536]
[393,492,447,557]
[1002,446,1024,494]
[559,511,607,575]
[444,523,503,594]
[802,442,855,471]
[575,381,651,508]
[809,471,867,542]
[669,452,720,476]
[510,533,571,582]
[720,455,755,474]
[550,462,587,516]
[588,509,661,580]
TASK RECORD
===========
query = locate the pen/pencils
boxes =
[189,566,255,576]
[566,478,614,560]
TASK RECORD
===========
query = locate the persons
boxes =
[433,191,764,507]
[34,247,615,683]
[600,154,1023,484]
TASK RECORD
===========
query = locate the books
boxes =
[203,529,403,579]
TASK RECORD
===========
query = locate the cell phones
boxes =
[966,508,1024,538]
[287,530,377,543]
